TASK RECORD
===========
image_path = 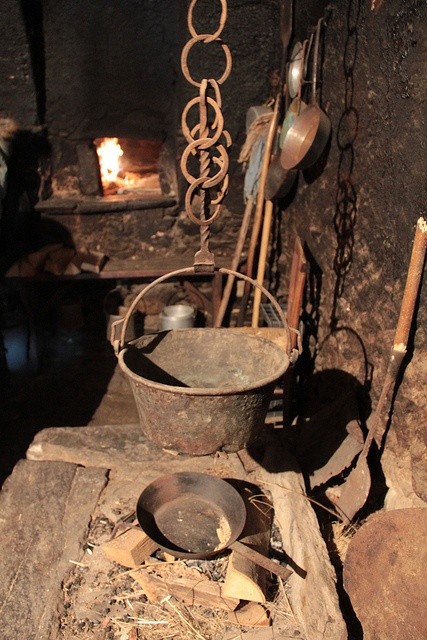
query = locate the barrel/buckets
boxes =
[110,265,297,457]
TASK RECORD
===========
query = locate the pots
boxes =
[136,471,293,581]
[280,18,331,169]
[288,41,307,97]
[246,96,276,137]
[264,83,298,200]
[280,41,306,149]
